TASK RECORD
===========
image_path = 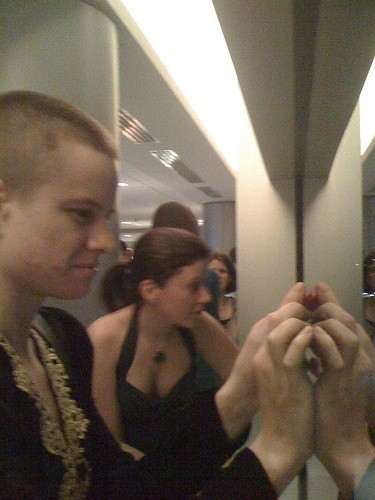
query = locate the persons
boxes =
[312,252,375,500]
[0,90,312,500]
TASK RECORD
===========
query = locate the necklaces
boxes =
[148,333,166,363]
